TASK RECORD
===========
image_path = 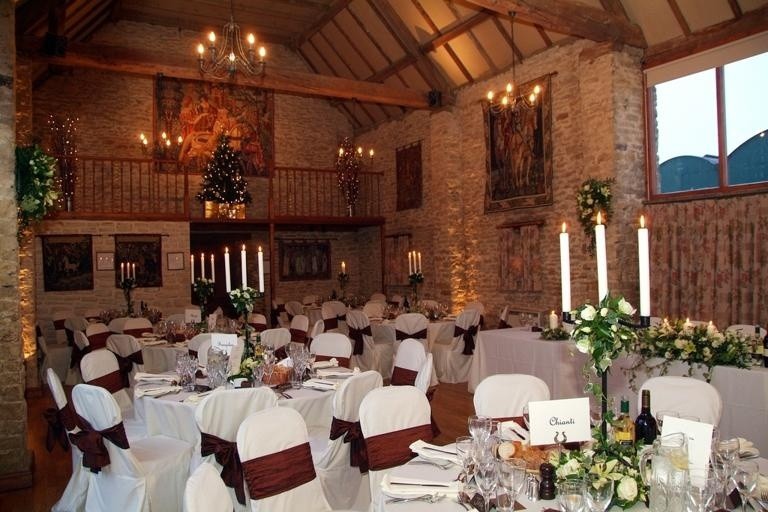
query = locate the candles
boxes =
[239,244,250,290]
[549,309,558,329]
[223,246,233,293]
[341,261,346,274]
[189,250,216,285]
[637,213,653,320]
[408,250,422,276]
[256,245,267,294]
[119,261,136,282]
[556,221,576,313]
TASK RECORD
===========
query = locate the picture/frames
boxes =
[151,78,277,180]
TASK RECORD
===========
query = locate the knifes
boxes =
[422,445,456,458]
[390,481,449,488]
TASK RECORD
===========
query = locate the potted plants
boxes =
[593,211,611,307]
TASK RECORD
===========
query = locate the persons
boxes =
[284,252,328,276]
[179,91,271,176]
[492,98,538,197]
[117,248,161,274]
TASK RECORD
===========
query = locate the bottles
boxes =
[748,326,767,370]
[613,394,637,450]
[525,472,541,504]
[634,388,656,440]
[764,330,767,368]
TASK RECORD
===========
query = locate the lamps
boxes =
[195,1,266,83]
[337,135,375,171]
[482,9,542,118]
[140,129,184,169]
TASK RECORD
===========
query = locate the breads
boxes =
[261,365,295,387]
[492,439,551,471]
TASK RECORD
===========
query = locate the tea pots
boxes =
[637,430,692,511]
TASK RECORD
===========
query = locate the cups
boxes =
[555,472,616,512]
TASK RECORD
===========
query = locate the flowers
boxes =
[651,314,751,373]
[572,298,639,377]
[553,443,648,508]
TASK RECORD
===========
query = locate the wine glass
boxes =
[318,293,449,321]
[456,413,525,512]
[151,318,237,342]
[655,409,768,511]
[515,309,533,332]
[99,302,162,325]
[139,343,355,404]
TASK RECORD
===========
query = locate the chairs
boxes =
[44,367,145,512]
[636,374,722,437]
[471,372,552,430]
[235,404,339,512]
[357,382,434,498]
[34,294,509,393]
[307,369,382,512]
[182,386,277,512]
[71,384,190,512]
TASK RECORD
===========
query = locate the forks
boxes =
[388,491,447,504]
[405,461,452,470]
